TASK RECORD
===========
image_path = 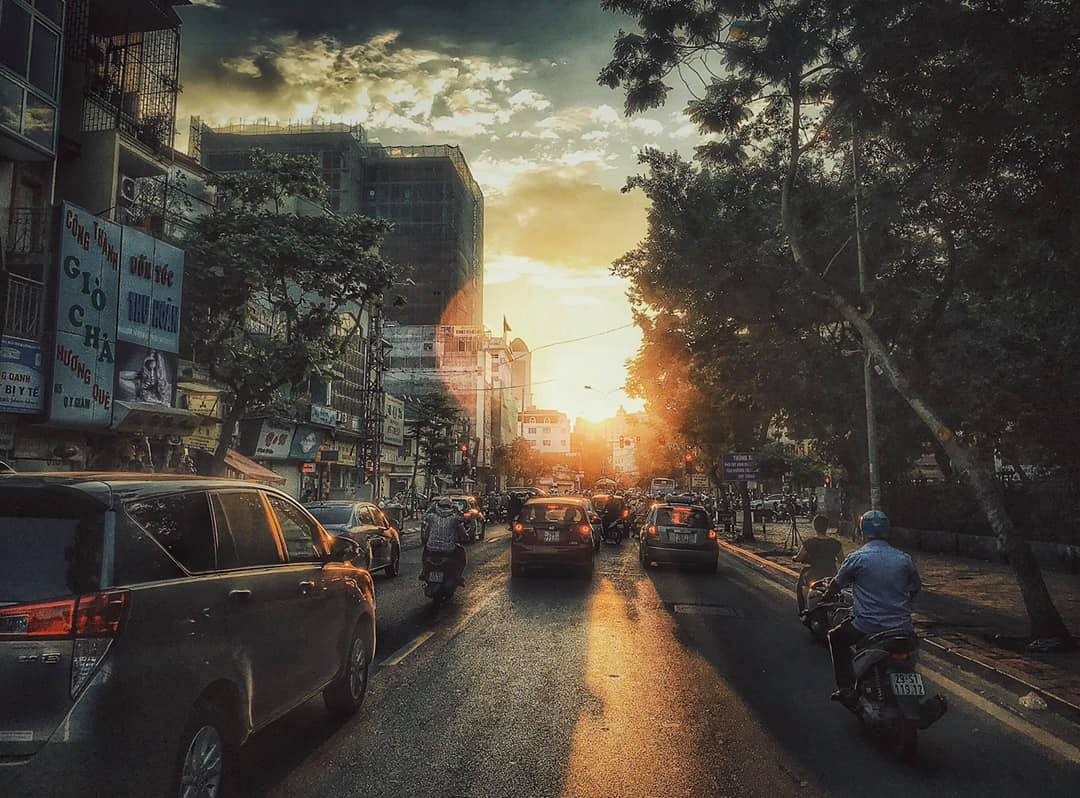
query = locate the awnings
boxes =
[178,381,223,417]
[208,446,287,489]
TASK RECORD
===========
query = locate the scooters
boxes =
[419,525,469,604]
[805,577,947,759]
[793,554,852,640]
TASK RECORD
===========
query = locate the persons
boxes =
[822,509,922,701]
[301,483,849,548]
[792,515,846,614]
[418,496,469,588]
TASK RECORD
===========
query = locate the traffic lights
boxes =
[620,435,640,450]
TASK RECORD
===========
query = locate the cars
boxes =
[422,497,487,544]
[750,494,801,513]
[509,497,597,579]
[638,504,721,571]
[277,500,400,577]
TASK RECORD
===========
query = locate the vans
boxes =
[508,486,548,497]
[0,473,377,797]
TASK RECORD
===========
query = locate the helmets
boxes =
[861,510,891,539]
[438,497,454,509]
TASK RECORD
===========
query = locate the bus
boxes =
[651,478,676,496]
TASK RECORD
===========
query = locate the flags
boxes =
[503,317,513,333]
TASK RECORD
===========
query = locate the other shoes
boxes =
[418,574,428,580]
[831,688,856,700]
[454,577,466,588]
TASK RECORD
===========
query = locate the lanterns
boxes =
[55,437,79,467]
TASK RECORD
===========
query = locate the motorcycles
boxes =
[599,510,624,545]
[483,500,507,526]
